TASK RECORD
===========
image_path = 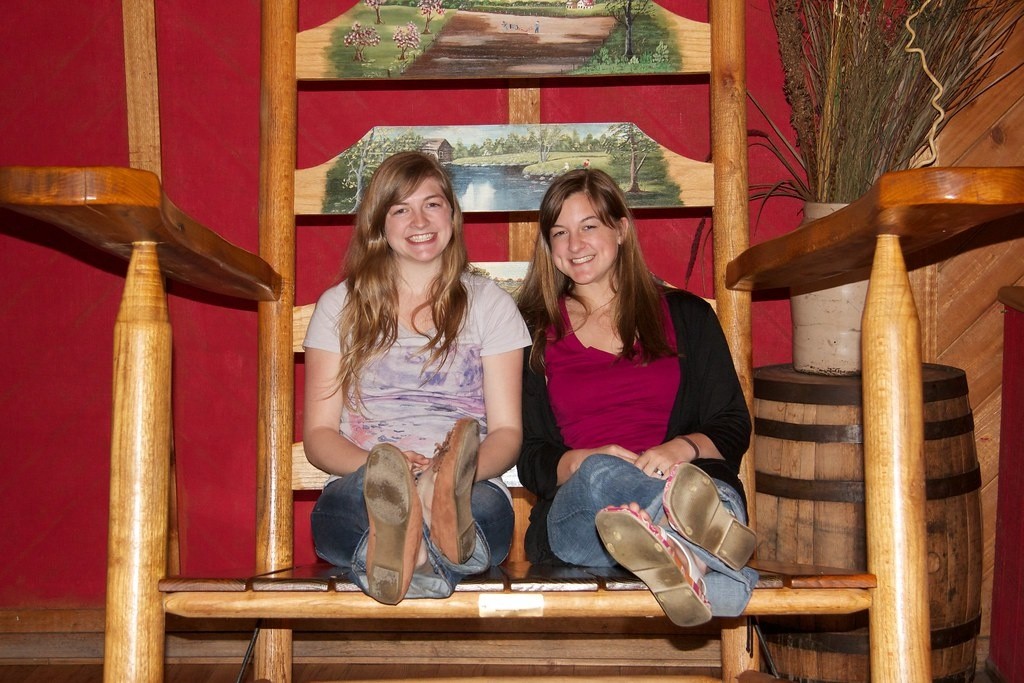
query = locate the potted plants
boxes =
[699,0,1023,378]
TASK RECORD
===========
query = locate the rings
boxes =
[653,467,665,478]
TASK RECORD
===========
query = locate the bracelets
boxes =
[675,435,699,460]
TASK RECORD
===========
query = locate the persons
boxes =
[301,151,532,608]
[510,167,758,627]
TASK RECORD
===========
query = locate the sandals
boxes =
[592,507,713,628]
[661,459,759,573]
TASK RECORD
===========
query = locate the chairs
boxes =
[0,1,1024,683]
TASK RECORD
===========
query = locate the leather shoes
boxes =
[361,442,423,606]
[428,418,481,566]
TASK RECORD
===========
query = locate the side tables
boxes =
[752,363,985,683]
